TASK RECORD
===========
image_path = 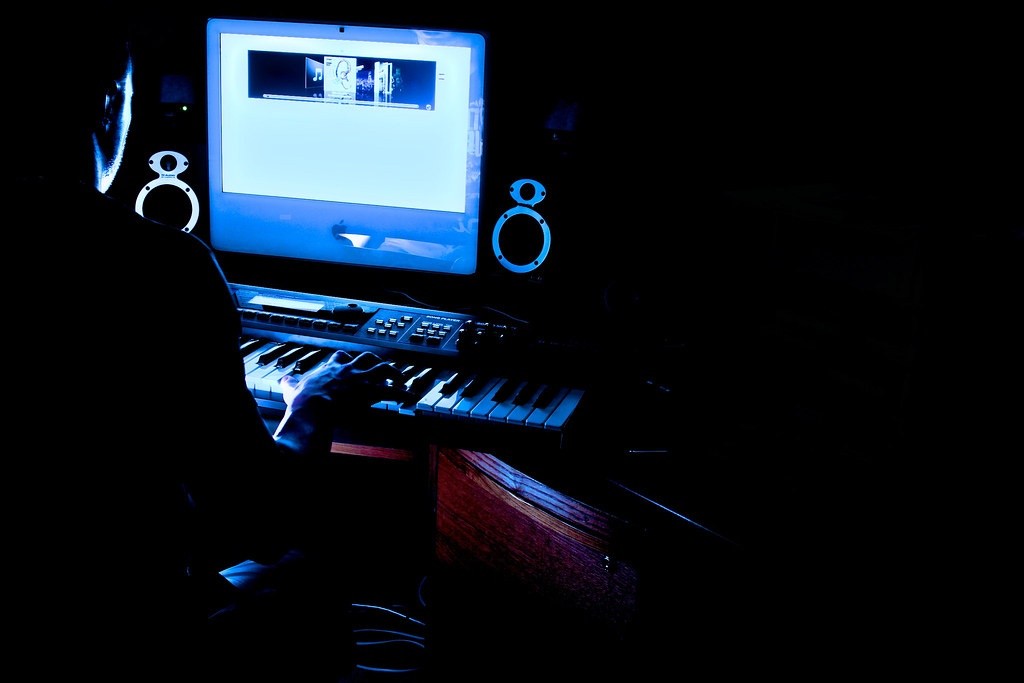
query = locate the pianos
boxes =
[219,275,611,449]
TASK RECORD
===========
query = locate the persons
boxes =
[0,0,415,559]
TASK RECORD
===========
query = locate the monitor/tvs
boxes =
[205,18,493,275]
